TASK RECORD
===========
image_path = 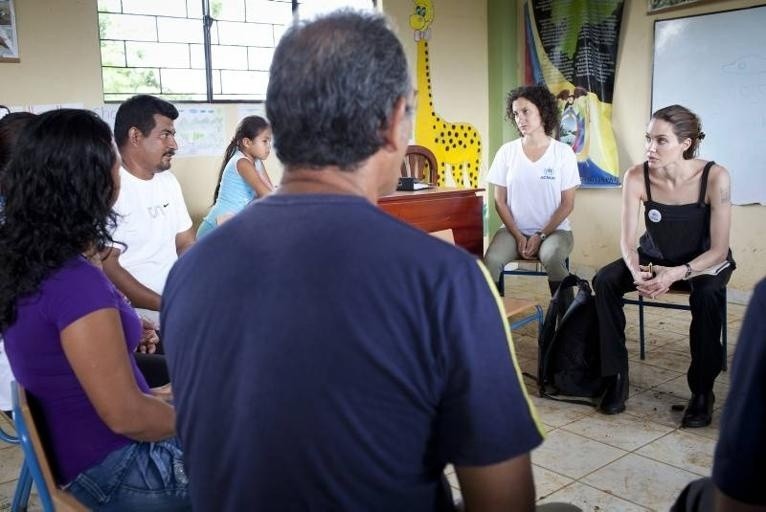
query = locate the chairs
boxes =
[1,380,89,511]
[399,145,437,185]
[499,254,729,374]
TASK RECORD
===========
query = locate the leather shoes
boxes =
[599,373,629,415]
[683,388,714,427]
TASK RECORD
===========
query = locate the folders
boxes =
[396,177,434,191]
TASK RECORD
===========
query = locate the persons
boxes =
[660,277,765,511]
[473,82,586,298]
[1,105,188,511]
[188,112,274,243]
[0,112,171,389]
[100,86,197,298]
[593,102,741,430]
[156,4,542,511]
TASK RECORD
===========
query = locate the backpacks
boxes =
[522,273,605,408]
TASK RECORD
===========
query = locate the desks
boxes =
[377,185,486,260]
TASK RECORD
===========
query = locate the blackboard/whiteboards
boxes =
[649,4,766,206]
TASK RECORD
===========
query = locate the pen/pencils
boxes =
[649,262,653,280]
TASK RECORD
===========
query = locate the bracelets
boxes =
[535,231,547,240]
[682,262,693,279]
